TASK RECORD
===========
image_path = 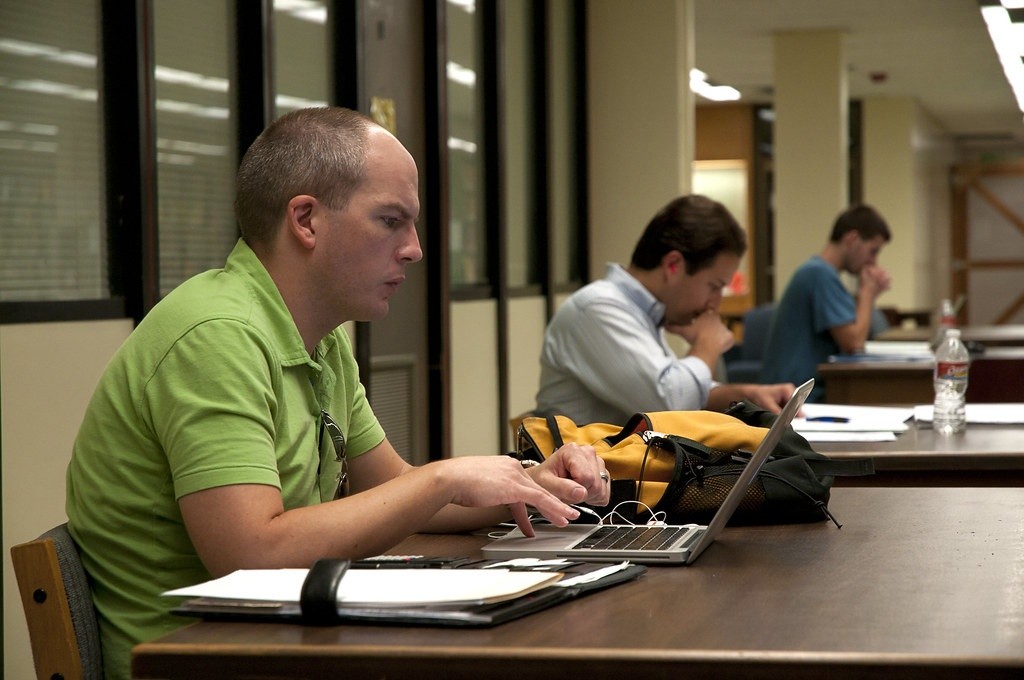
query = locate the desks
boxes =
[791,401,1024,488]
[131,484,1024,680]
[818,325,1024,403]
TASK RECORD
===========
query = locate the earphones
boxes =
[671,263,678,272]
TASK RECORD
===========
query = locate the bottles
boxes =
[941,297,956,329]
[934,329,969,434]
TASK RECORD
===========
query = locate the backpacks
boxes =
[507,399,875,529]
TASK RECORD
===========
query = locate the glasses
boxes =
[317,410,350,504]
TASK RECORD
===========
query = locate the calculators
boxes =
[351,555,471,569]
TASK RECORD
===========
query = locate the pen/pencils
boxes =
[807,417,850,422]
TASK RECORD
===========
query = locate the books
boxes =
[157,553,648,628]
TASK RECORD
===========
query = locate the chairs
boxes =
[10,524,109,680]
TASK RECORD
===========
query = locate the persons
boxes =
[534,194,804,428]
[64,108,610,680]
[762,204,892,404]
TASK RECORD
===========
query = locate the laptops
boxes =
[480,377,816,567]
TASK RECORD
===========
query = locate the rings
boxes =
[600,471,609,484]
